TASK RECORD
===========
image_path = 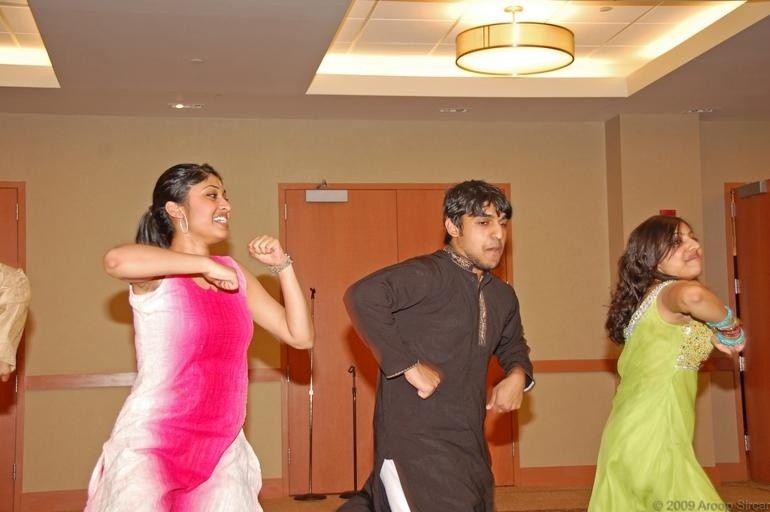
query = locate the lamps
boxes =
[454,6,583,77]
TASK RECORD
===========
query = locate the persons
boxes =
[585,216,747,512]
[80,159,315,512]
[0,260,31,384]
[343,179,537,512]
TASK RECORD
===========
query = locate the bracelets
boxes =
[706,305,745,345]
[272,255,292,273]
